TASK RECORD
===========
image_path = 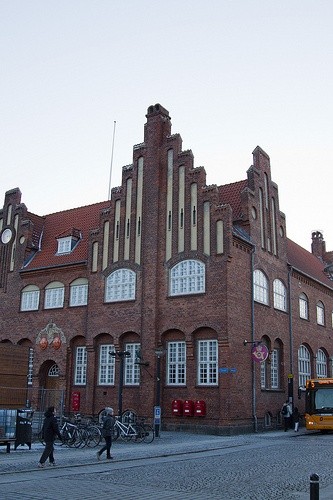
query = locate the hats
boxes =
[105,407,113,415]
[295,408,299,411]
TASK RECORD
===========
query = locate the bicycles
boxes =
[38,408,155,449]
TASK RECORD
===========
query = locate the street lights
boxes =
[108,350,132,438]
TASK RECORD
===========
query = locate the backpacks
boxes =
[281,405,288,415]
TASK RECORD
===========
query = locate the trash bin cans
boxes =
[15,408,35,449]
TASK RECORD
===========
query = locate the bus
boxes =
[298,377,333,435]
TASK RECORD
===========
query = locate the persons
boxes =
[96,407,117,461]
[280,400,292,432]
[38,407,63,468]
[292,407,303,432]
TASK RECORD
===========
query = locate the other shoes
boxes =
[106,456,114,459]
[284,430,287,432]
[297,429,298,432]
[38,462,46,467]
[96,452,101,461]
[295,431,296,432]
[49,461,58,466]
[291,427,294,430]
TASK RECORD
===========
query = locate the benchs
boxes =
[0,427,16,453]
